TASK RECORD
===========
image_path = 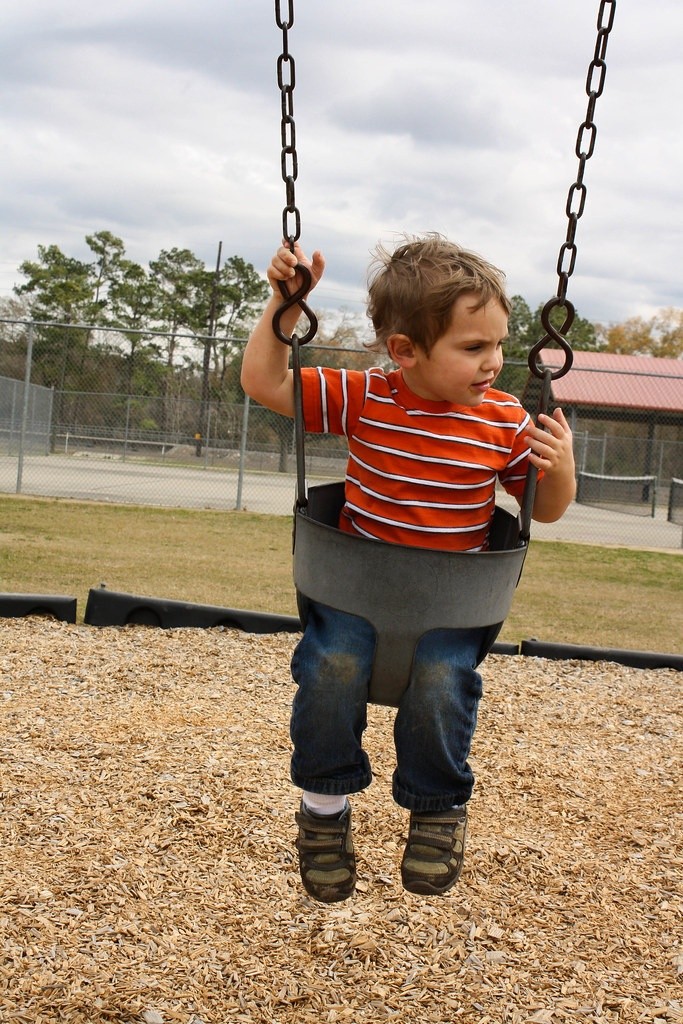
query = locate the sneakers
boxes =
[294,793,357,902]
[401,804,467,894]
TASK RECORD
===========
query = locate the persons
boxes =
[242,233,577,902]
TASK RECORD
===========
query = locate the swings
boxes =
[271,0,618,708]
